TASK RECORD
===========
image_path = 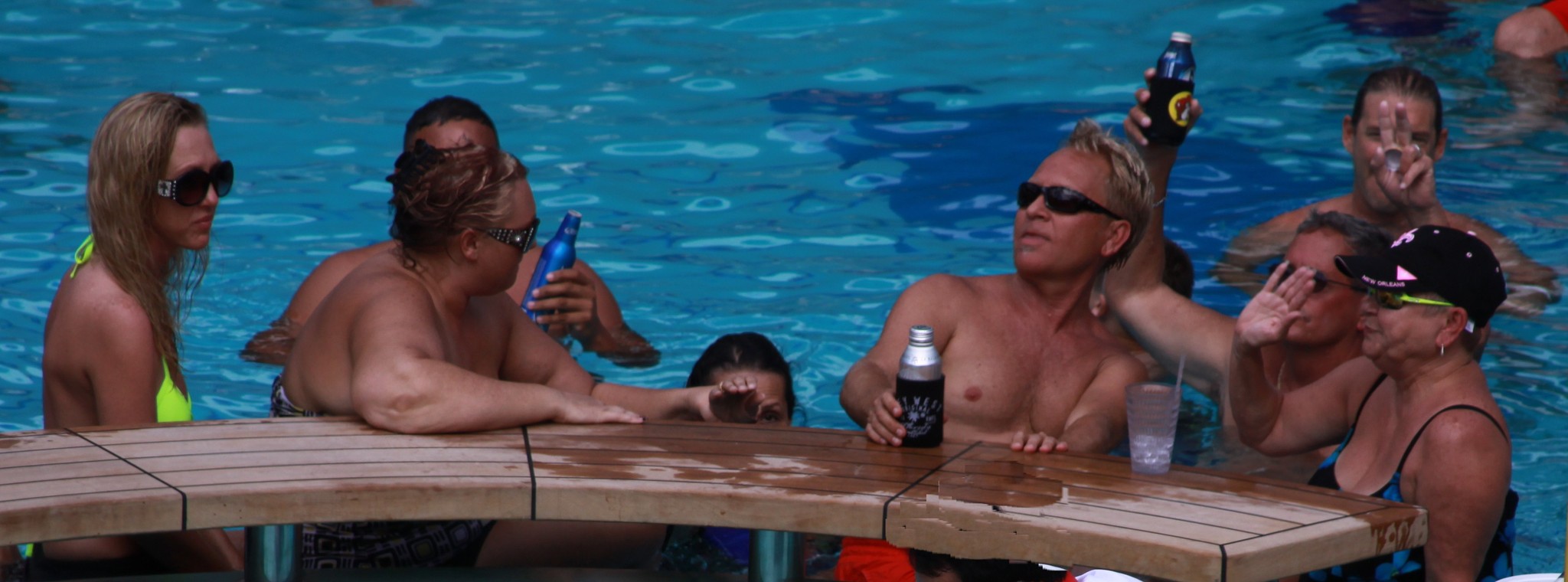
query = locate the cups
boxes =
[1125,381,1180,475]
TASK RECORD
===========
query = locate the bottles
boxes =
[895,325,943,449]
[519,209,583,332]
[1138,30,1197,147]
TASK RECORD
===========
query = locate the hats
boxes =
[1335,224,1507,326]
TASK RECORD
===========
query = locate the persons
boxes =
[834,118,1155,582]
[906,548,1143,582]
[267,135,726,582]
[1229,225,1512,582]
[29,91,245,576]
[244,95,653,355]
[1089,239,1217,404]
[1228,65,1526,267]
[1102,66,1491,483]
[1494,0,1568,58]
[653,333,842,582]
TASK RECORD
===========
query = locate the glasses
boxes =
[470,219,539,254]
[1270,261,1370,293]
[1367,285,1455,309]
[151,161,234,207]
[1018,181,1123,220]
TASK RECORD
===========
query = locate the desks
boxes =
[0,411,1437,582]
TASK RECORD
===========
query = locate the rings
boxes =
[1413,144,1421,158]
